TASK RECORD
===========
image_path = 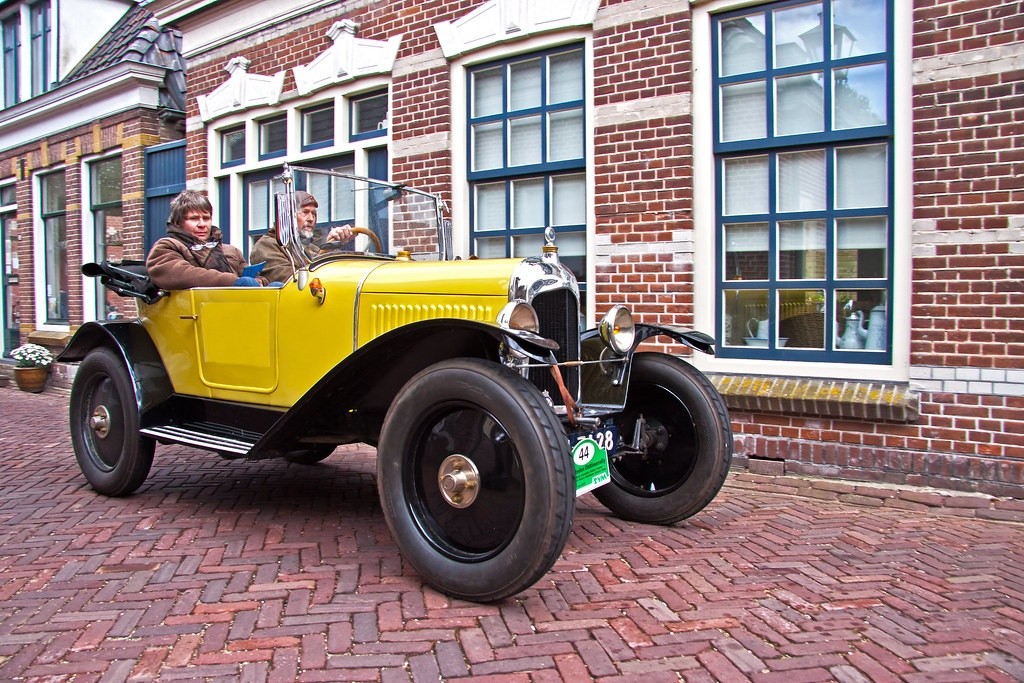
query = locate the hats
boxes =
[295,191,318,209]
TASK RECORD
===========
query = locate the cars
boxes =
[55,156,731,606]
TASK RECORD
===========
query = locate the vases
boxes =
[14,366,48,393]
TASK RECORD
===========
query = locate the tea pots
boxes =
[747,318,770,338]
[856,301,886,351]
[833,311,865,349]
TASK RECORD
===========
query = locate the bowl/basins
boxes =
[744,337,790,347]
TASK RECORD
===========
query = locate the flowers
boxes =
[9,344,54,369]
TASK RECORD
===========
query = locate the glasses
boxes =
[297,209,316,218]
[190,242,218,250]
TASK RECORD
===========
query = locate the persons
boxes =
[249,190,358,287]
[146,189,286,288]
